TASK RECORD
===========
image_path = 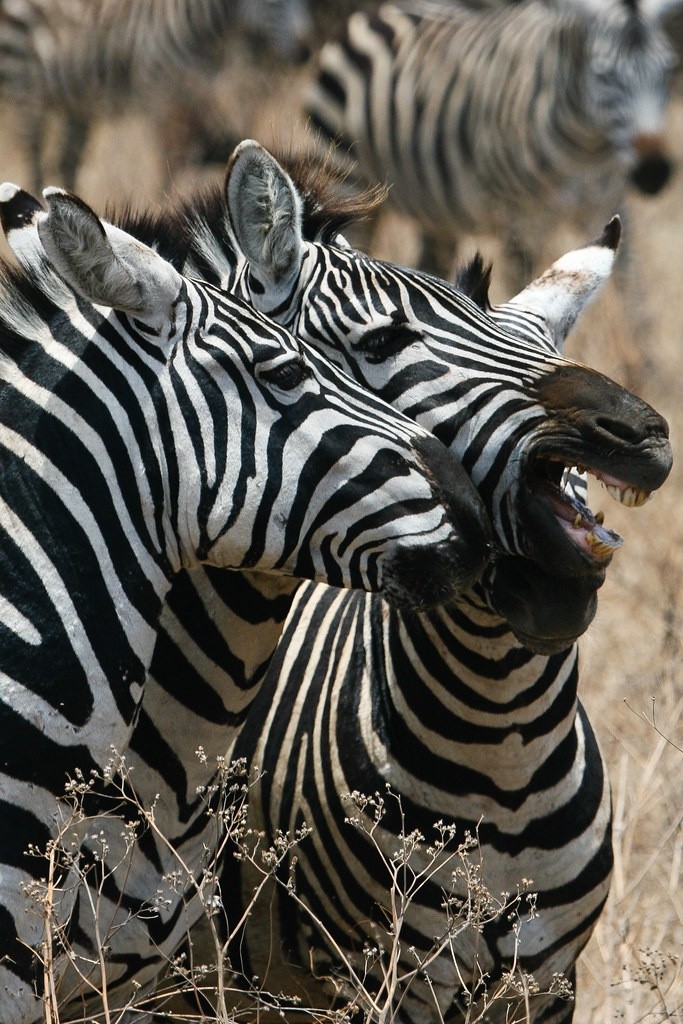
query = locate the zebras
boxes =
[0,1,683,1024]
